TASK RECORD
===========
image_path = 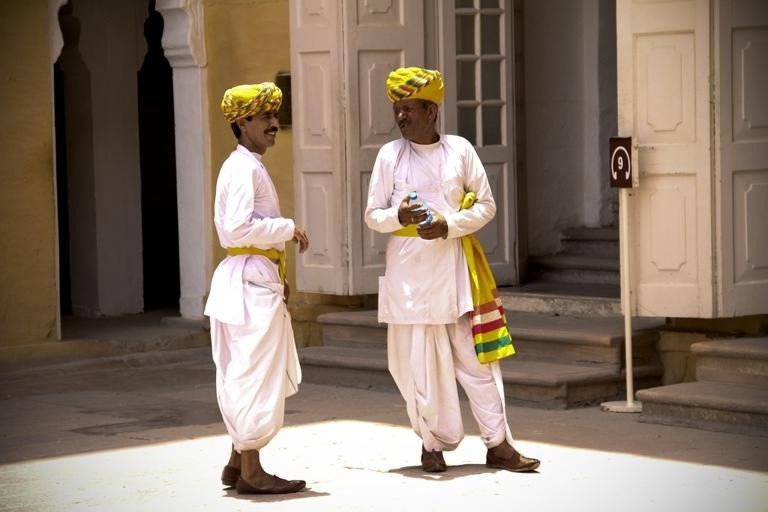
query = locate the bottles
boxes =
[408,190,433,228]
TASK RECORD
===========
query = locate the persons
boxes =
[204,82,309,495]
[364,67,540,473]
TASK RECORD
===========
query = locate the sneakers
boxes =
[222,465,306,495]
[421,443,446,473]
[486,448,542,471]
